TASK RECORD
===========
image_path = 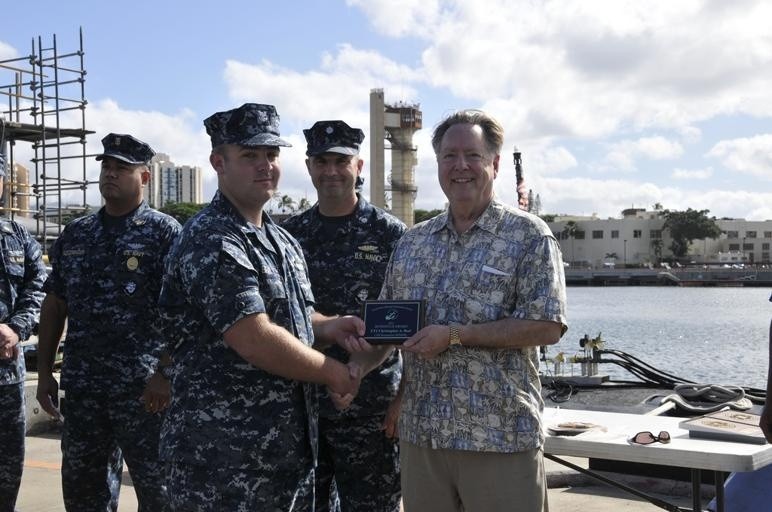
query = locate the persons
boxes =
[148,102,388,510]
[35,132,191,511]
[326,107,569,511]
[273,119,410,512]
[0,156,49,511]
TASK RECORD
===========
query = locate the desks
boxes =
[542,407,772,510]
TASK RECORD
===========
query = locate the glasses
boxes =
[627,430,670,445]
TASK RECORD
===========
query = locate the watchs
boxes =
[447,325,464,353]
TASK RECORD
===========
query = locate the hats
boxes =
[96,132,156,163]
[204,103,292,148]
[304,121,365,155]
